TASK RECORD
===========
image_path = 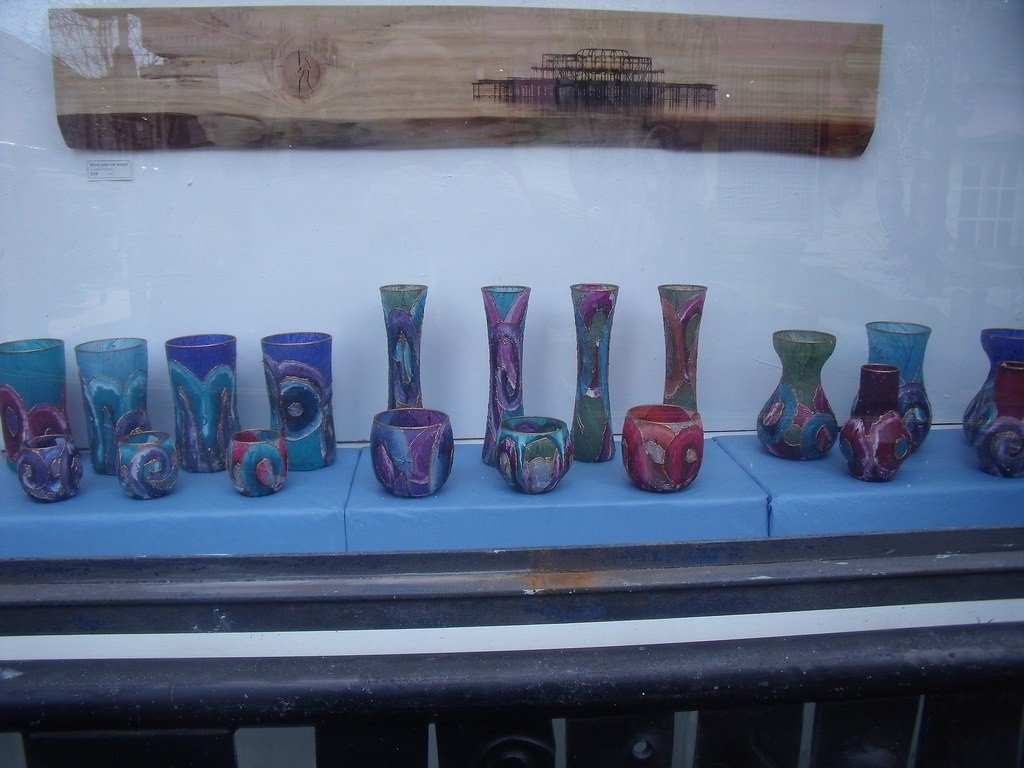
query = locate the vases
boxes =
[757,329,838,461]
[866,321,932,451]
[74,338,147,474]
[165,334,239,473]
[570,283,620,462]
[380,284,428,410]
[261,332,336,471]
[482,286,531,467]
[961,327,1024,478]
[0,338,70,471]
[840,363,911,482]
[659,284,709,410]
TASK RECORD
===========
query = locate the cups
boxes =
[260,332,337,471]
[621,404,703,492]
[494,416,573,494]
[17,434,83,502]
[74,338,150,476]
[226,429,287,497]
[165,334,240,473]
[370,408,454,497]
[0,338,73,473]
[117,431,178,500]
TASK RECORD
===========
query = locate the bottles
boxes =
[481,285,531,467]
[850,321,932,453]
[570,283,619,463]
[962,328,1024,478]
[839,364,912,483]
[379,284,428,410]
[757,330,838,460]
[657,284,708,410]
